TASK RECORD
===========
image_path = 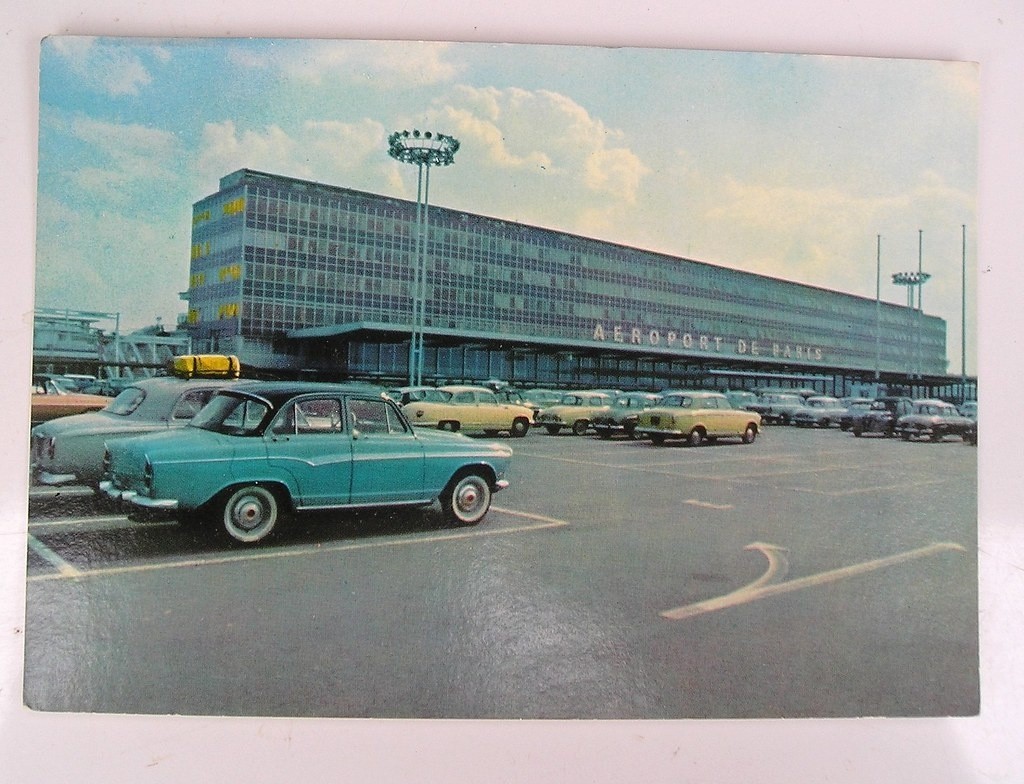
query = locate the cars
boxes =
[593,390,664,441]
[795,395,849,429]
[535,391,611,437]
[895,400,977,442]
[747,392,806,427]
[102,380,512,549]
[853,396,914,439]
[31,374,342,513]
[389,385,544,438]
[635,389,761,447]
